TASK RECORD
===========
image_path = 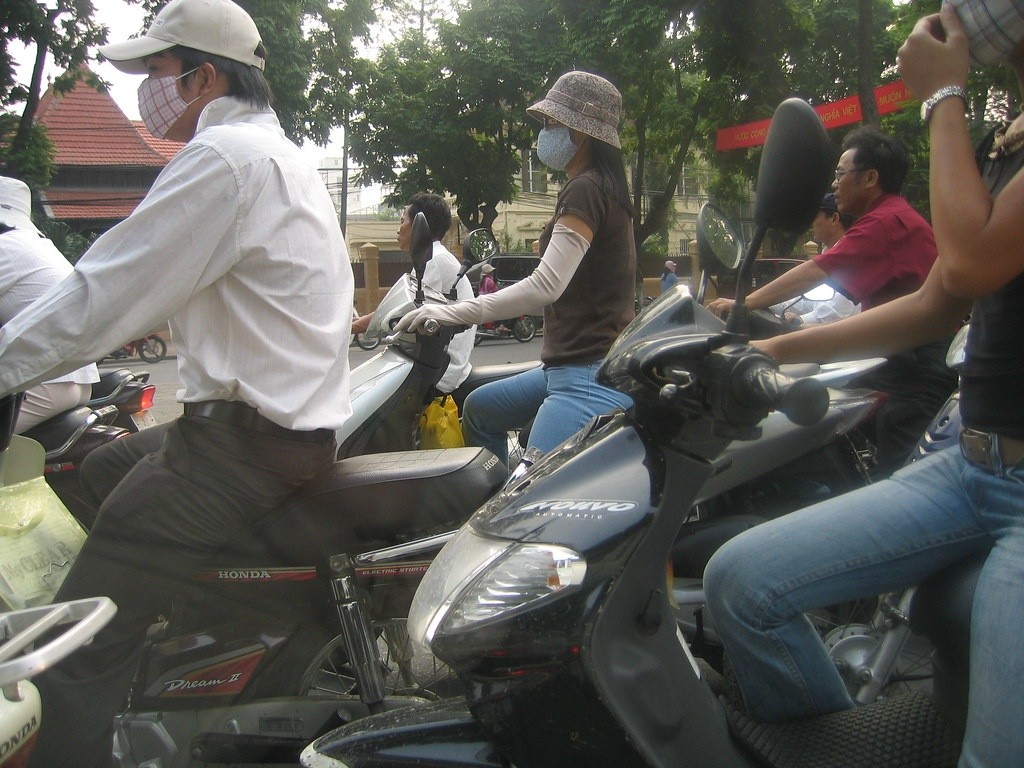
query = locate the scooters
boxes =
[0,211,970,768]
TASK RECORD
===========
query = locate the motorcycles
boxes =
[300,96,994,768]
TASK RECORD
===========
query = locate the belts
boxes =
[960,428,1024,467]
[184,400,330,442]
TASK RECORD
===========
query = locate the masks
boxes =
[537,127,585,172]
[137,67,203,139]
[941,0,1024,69]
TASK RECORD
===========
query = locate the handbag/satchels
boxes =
[418,393,464,449]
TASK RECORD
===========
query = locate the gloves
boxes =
[392,224,591,336]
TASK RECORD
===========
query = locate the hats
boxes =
[0,176,46,238]
[525,70,623,152]
[818,192,836,209]
[665,261,677,273]
[98,0,266,75]
[480,264,496,278]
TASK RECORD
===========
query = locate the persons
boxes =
[0,176,100,434]
[0,0,355,768]
[351,194,478,405]
[479,264,500,295]
[702,0,1024,768]
[659,261,678,291]
[393,70,636,470]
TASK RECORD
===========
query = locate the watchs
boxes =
[920,85,969,122]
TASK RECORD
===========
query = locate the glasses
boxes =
[541,117,558,130]
[834,169,865,181]
